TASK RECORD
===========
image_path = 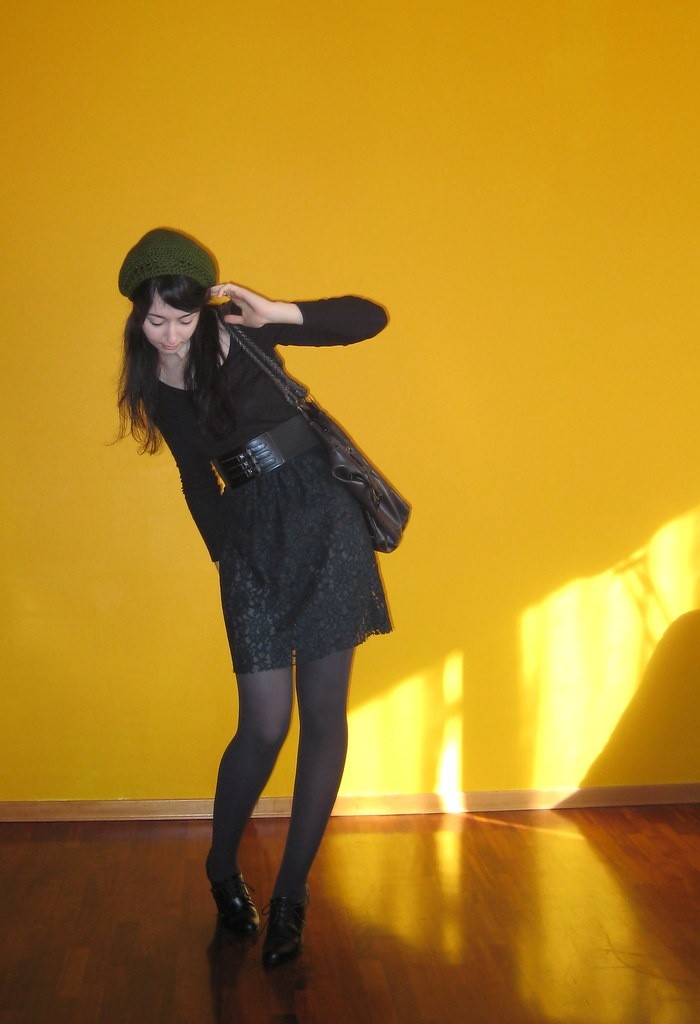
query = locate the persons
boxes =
[108,226,392,971]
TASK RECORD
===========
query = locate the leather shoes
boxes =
[261,883,310,966]
[206,858,260,933]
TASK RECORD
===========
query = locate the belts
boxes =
[213,413,321,488]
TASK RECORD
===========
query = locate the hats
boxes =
[118,228,220,299]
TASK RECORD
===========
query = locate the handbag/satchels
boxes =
[209,305,413,554]
